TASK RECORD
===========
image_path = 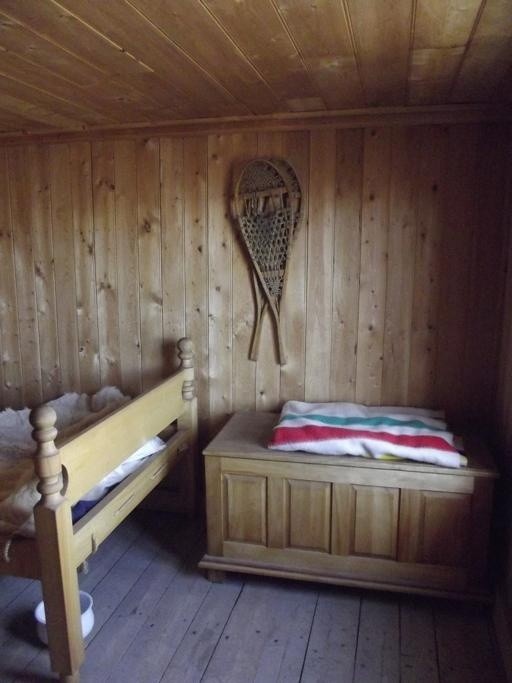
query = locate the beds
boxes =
[0,337,199,683]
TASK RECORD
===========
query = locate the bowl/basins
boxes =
[34,589,95,647]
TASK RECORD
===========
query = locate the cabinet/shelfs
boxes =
[198,409,512,611]
